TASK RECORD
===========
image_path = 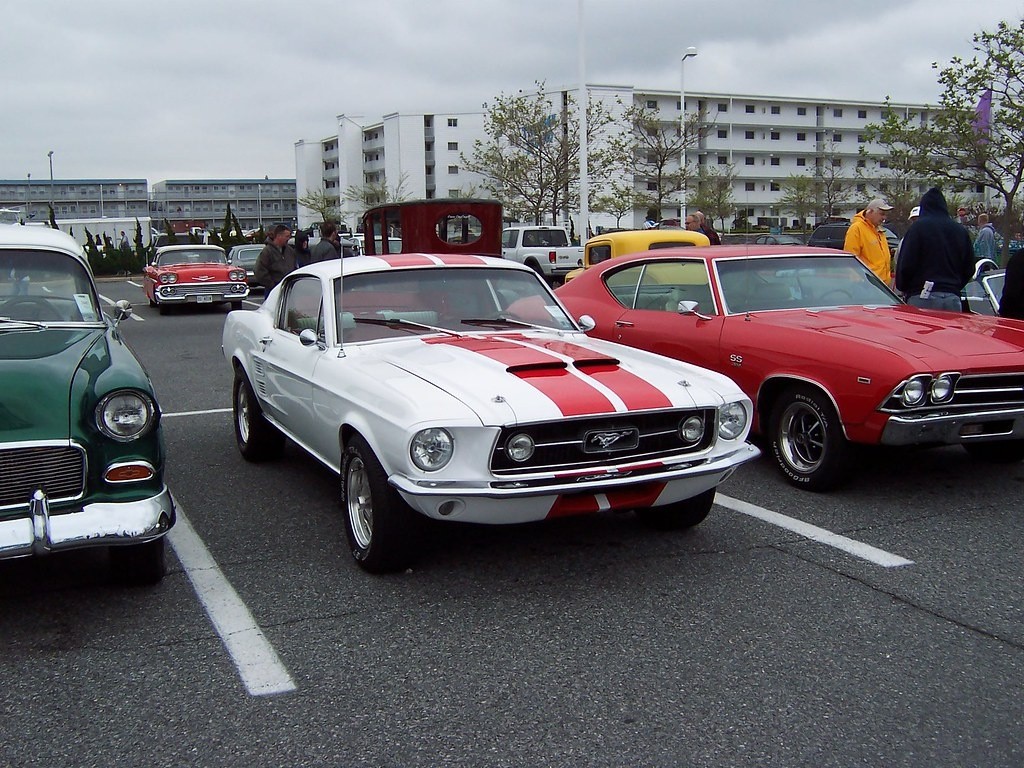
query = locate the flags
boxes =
[968,89,991,146]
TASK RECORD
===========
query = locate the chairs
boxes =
[176,255,188,264]
[666,288,716,315]
[754,284,792,302]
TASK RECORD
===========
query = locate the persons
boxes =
[9,264,54,298]
[643,210,722,245]
[843,197,896,288]
[94,234,103,245]
[996,230,1024,320]
[973,213,996,264]
[253,222,354,300]
[893,187,976,314]
[108,237,114,249]
[119,230,129,250]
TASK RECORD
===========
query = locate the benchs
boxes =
[618,294,667,309]
[0,297,77,322]
[292,285,447,334]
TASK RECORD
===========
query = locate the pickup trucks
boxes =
[501,226,586,290]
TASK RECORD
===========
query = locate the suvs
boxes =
[806,223,900,259]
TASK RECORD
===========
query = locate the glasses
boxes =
[685,221,696,225]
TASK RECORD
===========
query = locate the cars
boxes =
[0,226,178,590]
[222,251,762,576]
[150,222,364,247]
[959,256,1006,317]
[350,237,403,256]
[143,244,249,317]
[507,245,1023,490]
[227,244,267,291]
[745,235,804,245]
[564,230,711,314]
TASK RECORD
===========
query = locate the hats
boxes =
[908,205,920,220]
[642,220,660,229]
[867,199,895,210]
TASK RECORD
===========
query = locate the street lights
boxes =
[47,150,54,211]
[679,47,698,229]
